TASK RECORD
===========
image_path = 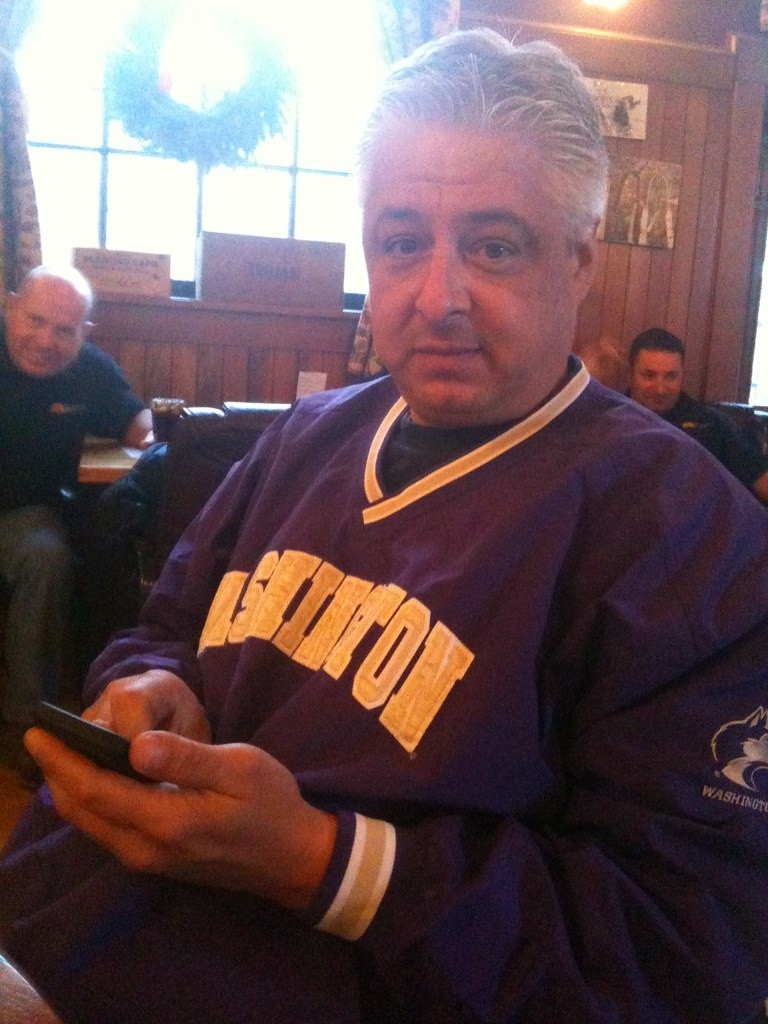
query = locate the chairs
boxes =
[134,400,290,615]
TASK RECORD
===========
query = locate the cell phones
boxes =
[34,700,145,783]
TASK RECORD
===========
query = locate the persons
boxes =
[0,262,160,783]
[620,323,768,508]
[0,24,767,1024]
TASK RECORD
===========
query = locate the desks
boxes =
[78,433,145,482]
